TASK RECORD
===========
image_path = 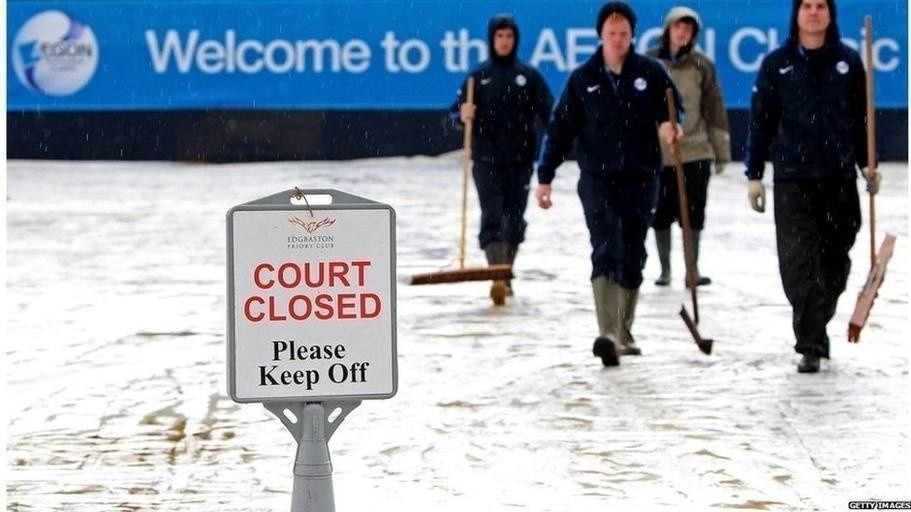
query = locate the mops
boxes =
[664,88,714,355]
[406,76,518,286]
[846,13,897,342]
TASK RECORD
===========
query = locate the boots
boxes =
[594,276,640,365]
[485,242,518,304]
[656,228,671,285]
[686,230,710,287]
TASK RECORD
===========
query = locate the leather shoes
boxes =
[798,354,820,372]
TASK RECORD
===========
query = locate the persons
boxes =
[640,4,735,293]
[533,2,686,368]
[446,10,553,307]
[743,0,884,374]
